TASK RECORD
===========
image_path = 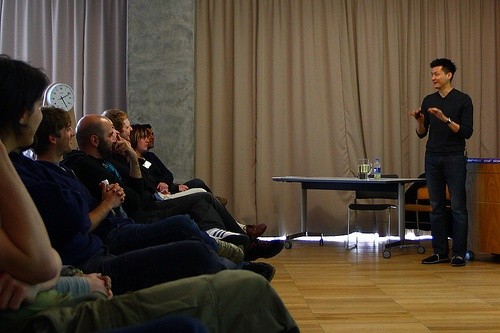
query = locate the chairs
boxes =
[405,173,452,245]
[345,173,399,251]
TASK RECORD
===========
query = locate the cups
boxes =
[358,159,373,179]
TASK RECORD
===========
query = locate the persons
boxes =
[0,53,300,333]
[413,58,474,266]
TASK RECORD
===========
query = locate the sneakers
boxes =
[244,237,284,261]
[214,238,244,264]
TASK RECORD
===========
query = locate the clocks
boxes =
[46,82,74,112]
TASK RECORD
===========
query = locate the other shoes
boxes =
[243,223,267,238]
[215,195,228,206]
[243,261,276,283]
[422,254,451,264]
[451,256,466,266]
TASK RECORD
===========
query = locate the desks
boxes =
[465,163,500,256]
[271,175,430,259]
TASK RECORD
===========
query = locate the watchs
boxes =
[446,118,451,124]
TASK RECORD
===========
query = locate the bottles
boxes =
[374,159,381,180]
